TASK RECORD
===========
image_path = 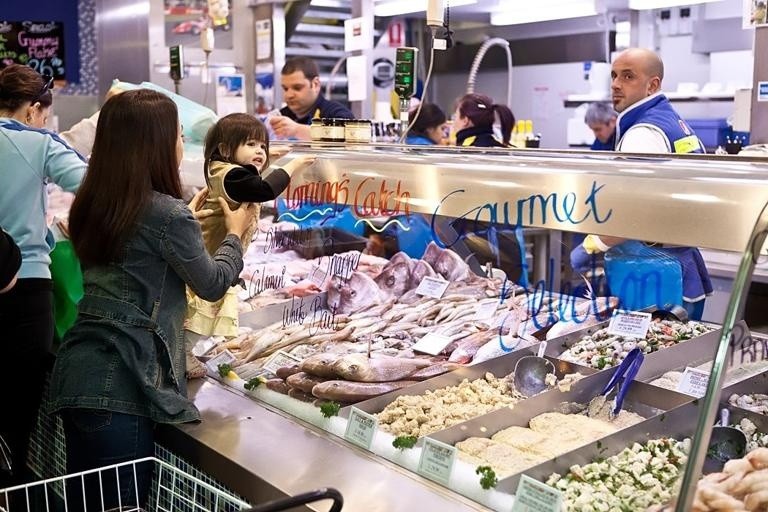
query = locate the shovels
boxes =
[512,339,555,397]
[701,407,747,476]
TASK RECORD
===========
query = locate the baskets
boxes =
[726,129,750,148]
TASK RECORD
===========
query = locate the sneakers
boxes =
[186,352,207,379]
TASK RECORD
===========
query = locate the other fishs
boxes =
[207,239,619,407]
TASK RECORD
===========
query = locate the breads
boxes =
[643,445,768,511]
[452,395,625,477]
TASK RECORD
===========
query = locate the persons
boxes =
[451,93,530,290]
[262,56,366,238]
[0,65,90,511]
[47,85,126,225]
[182,113,316,380]
[584,101,618,150]
[395,102,448,261]
[568,47,715,322]
[45,88,258,512]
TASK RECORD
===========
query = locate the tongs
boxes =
[588,347,644,422]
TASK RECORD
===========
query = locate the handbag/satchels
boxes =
[51,219,84,342]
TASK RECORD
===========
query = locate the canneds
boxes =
[310,116,371,144]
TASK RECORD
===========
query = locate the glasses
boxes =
[30,74,54,106]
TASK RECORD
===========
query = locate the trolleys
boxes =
[1,456,342,512]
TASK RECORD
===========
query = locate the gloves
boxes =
[570,234,609,271]
